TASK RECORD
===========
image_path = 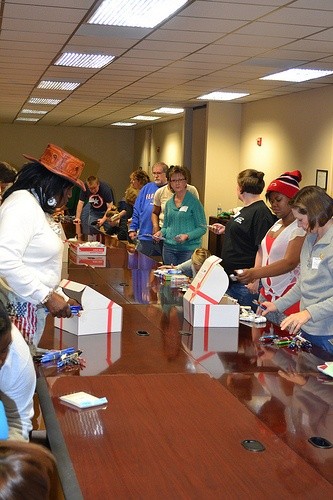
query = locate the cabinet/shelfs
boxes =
[208,216,233,261]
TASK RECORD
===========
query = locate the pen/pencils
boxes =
[252,300,271,315]
[259,333,313,353]
[45,305,80,313]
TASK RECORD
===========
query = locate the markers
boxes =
[37,346,82,367]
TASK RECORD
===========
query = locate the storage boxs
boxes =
[181,318,239,379]
[69,240,107,255]
[69,250,107,268]
[53,278,123,336]
[182,255,241,328]
[54,327,122,376]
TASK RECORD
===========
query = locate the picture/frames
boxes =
[316,170,328,190]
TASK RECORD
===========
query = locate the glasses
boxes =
[171,178,186,183]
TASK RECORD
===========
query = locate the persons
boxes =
[252,327,333,405]
[210,169,275,313]
[235,170,307,325]
[73,170,149,244]
[128,162,168,256]
[75,223,120,247]
[256,185,333,355]
[0,145,86,500]
[152,166,200,236]
[152,165,208,277]
[157,249,211,279]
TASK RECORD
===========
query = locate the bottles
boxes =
[217,202,222,215]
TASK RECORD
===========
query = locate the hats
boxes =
[22,143,86,192]
[267,170,302,199]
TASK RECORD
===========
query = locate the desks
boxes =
[29,219,333,500]
